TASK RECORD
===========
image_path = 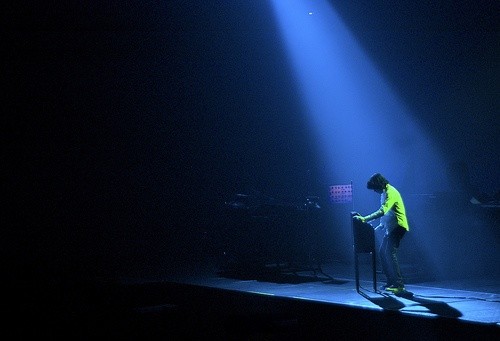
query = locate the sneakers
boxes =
[380,284,390,290]
[385,285,405,291]
